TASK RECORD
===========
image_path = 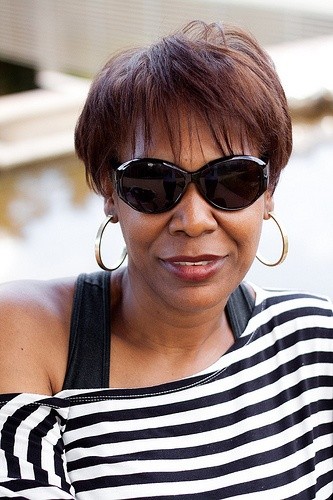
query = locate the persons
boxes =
[0,18,333,500]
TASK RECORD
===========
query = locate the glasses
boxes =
[110,154,272,215]
